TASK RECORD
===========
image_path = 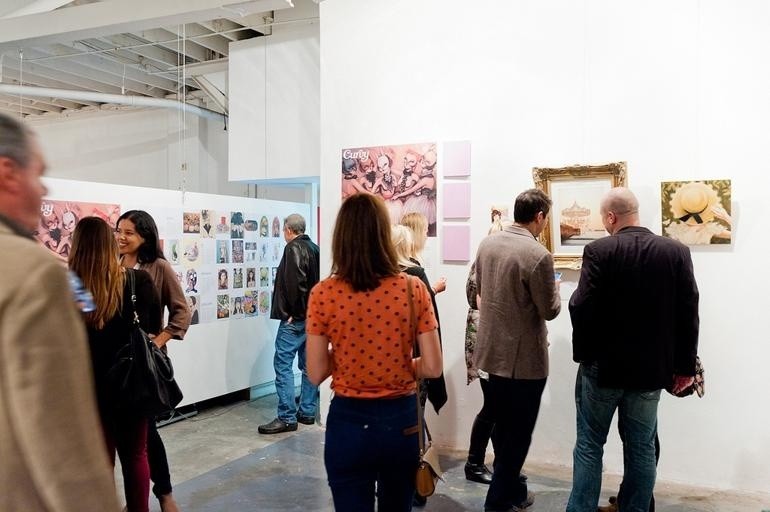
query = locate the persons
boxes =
[343,148,437,223]
[568,184,696,509]
[1,110,127,512]
[68,217,160,512]
[40,210,78,258]
[112,210,193,510]
[668,184,732,245]
[258,215,321,435]
[390,213,448,508]
[474,189,560,512]
[463,248,529,483]
[308,194,446,512]
[177,209,281,327]
[609,336,706,510]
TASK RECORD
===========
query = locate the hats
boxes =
[669,181,722,226]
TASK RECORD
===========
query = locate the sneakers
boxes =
[517,490,535,509]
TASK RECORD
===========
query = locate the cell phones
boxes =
[555,272,562,280]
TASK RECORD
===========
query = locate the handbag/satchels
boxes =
[416,444,446,497]
[667,354,704,398]
[122,268,183,416]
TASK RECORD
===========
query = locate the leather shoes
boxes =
[597,502,618,512]
[465,460,494,483]
[258,417,298,434]
[296,410,314,424]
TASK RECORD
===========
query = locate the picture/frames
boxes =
[529,160,628,269]
